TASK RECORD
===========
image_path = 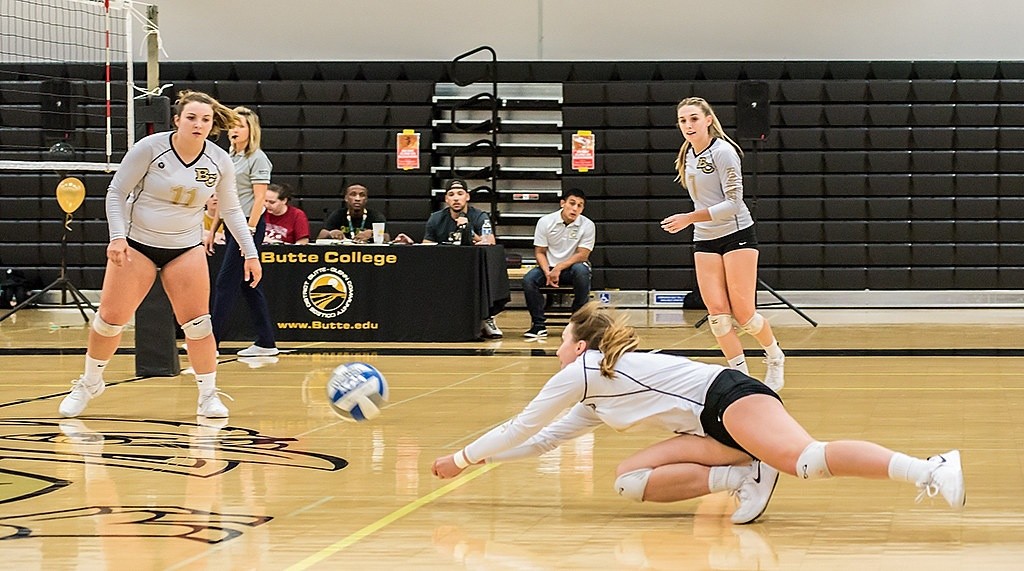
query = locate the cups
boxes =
[372,223,385,244]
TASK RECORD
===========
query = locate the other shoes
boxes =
[59,416,105,457]
[471,340,502,356]
[238,356,279,368]
[188,416,229,454]
[180,359,221,375]
[731,526,777,561]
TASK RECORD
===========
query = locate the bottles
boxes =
[482,220,491,244]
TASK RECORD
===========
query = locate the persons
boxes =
[432,296,968,524]
[57,93,263,420]
[203,106,597,357]
[662,97,785,394]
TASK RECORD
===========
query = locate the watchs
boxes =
[247,225,257,235]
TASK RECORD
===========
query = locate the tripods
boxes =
[0,210,98,322]
[695,277,818,327]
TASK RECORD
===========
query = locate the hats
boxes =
[446,178,468,192]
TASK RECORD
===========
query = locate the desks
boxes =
[176,240,515,347]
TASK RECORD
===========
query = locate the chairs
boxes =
[540,284,574,328]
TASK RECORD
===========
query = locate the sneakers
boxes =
[58,373,105,418]
[523,325,548,338]
[181,343,219,358]
[237,344,279,357]
[914,449,966,507]
[196,388,234,418]
[729,460,780,525]
[482,316,503,338]
[761,352,785,392]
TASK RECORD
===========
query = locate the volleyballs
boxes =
[326,361,389,424]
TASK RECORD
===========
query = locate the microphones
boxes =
[458,212,466,230]
